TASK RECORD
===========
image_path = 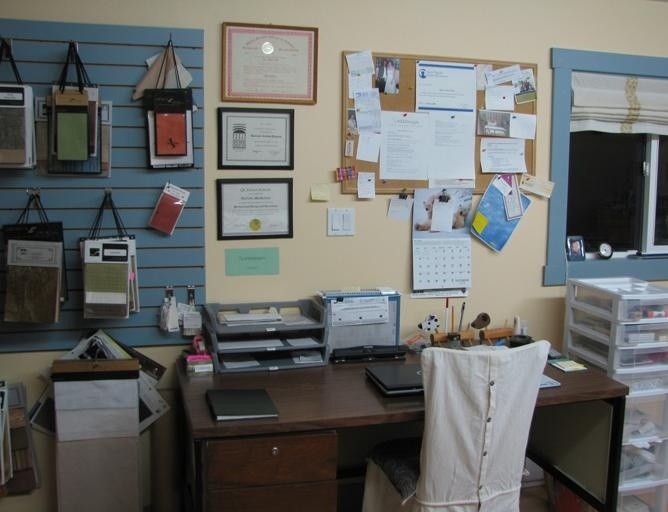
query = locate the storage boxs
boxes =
[54,429,153,512]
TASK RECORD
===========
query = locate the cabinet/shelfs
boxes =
[561,275,667,511]
[200,294,334,373]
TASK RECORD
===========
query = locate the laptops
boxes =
[365,362,424,395]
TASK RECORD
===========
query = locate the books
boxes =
[204,386,279,421]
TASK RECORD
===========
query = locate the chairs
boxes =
[363,337,553,511]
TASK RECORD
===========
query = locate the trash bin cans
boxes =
[336,468,364,512]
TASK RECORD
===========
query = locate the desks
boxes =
[170,338,630,510]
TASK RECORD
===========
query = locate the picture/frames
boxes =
[565,234,585,263]
[215,176,294,243]
[219,20,320,108]
[215,106,296,171]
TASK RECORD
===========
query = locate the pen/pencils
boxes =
[451,306,455,333]
[458,302,465,332]
[444,297,448,334]
